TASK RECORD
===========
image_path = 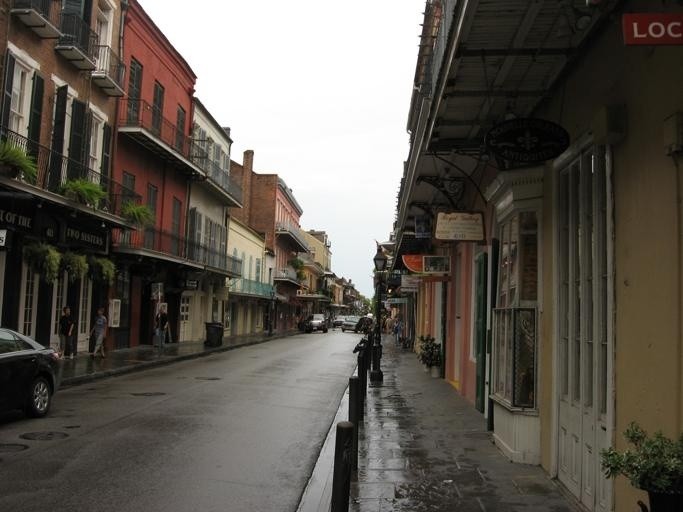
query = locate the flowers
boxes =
[414,336,440,367]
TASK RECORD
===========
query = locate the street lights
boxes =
[265,286,276,337]
[365,243,389,383]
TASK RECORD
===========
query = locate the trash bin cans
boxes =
[204,322,223,347]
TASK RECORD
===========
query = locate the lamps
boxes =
[373,242,396,271]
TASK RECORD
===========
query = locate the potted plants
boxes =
[598,420,682,512]
[0,134,39,185]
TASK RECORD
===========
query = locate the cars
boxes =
[296,306,373,337]
[1,325,63,422]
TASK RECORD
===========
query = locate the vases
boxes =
[422,362,440,379]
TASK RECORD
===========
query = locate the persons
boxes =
[88,308,107,358]
[59,307,75,360]
[153,305,169,347]
